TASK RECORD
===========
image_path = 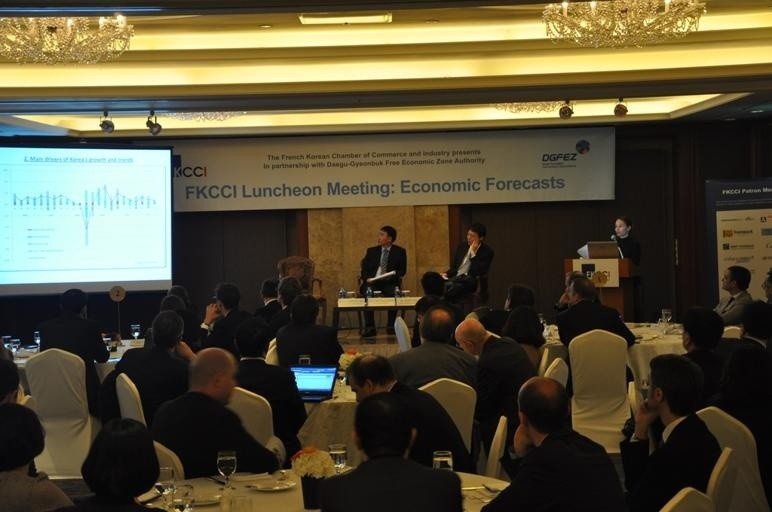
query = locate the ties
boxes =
[725,297,734,307]
[381,249,389,275]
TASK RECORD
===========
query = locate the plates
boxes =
[246,482,296,491]
[174,496,220,505]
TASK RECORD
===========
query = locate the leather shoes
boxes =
[359,326,377,337]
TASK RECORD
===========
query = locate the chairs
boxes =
[568,329,629,453]
[544,357,568,390]
[296,399,357,450]
[225,384,286,467]
[707,444,765,510]
[658,487,713,512]
[279,255,328,326]
[419,377,478,453]
[151,439,186,483]
[21,348,93,481]
[113,370,150,430]
[538,347,549,375]
[661,404,760,476]
[486,415,509,480]
[356,276,402,335]
[393,316,417,352]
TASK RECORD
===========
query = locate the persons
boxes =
[2,266,772,512]
[439,224,495,304]
[612,217,641,267]
[358,225,407,337]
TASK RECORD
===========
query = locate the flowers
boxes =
[338,347,364,371]
[291,448,337,478]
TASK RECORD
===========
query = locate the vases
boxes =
[297,475,320,512]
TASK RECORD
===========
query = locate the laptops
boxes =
[289,364,339,402]
[586,241,618,260]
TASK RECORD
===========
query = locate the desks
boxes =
[330,304,416,338]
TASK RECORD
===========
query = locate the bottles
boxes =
[339,288,346,297]
[394,286,400,297]
[366,287,373,297]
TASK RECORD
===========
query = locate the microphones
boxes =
[610,234,624,258]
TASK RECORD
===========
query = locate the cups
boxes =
[217,451,237,490]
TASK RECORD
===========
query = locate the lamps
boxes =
[146,111,161,134]
[614,99,628,115]
[98,112,114,132]
[560,101,574,119]
[1,14,136,68]
[541,1,707,50]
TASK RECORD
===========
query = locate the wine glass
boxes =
[130,324,140,341]
[2,336,20,361]
[172,485,195,512]
[154,467,176,508]
[33,331,40,351]
[103,338,113,352]
[432,451,453,471]
[327,444,348,474]
[657,308,670,339]
